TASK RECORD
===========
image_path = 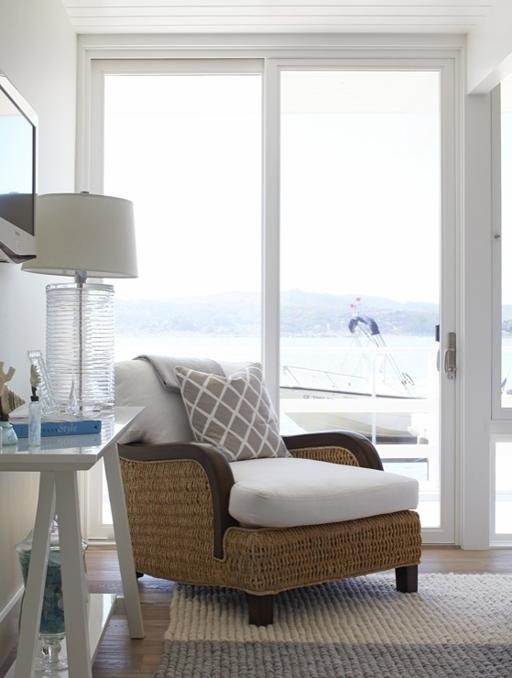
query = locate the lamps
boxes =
[19,191,139,422]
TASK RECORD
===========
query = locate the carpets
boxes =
[157,573,512,678]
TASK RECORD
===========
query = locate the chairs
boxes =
[115,355,423,627]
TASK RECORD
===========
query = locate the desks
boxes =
[1,403,147,678]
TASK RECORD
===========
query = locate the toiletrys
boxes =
[29,387,42,447]
[0,414,19,445]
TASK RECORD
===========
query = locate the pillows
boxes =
[178,363,289,460]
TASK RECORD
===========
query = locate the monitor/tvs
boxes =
[0,74,40,264]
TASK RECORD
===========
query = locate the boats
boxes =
[279,296,512,444]
